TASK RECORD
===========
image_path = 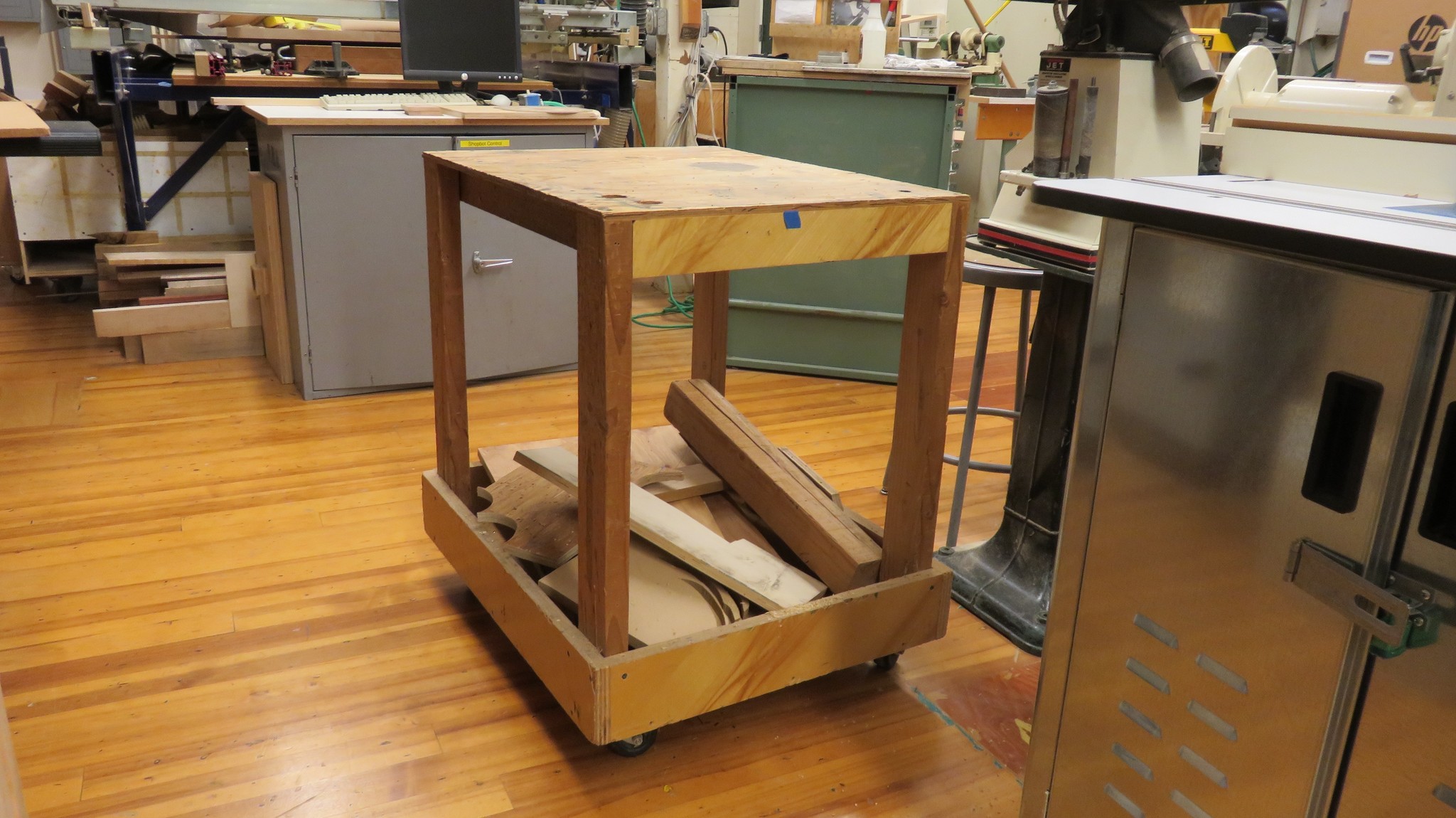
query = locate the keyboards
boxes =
[319,91,475,112]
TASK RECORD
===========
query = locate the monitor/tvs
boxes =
[398,0,523,83]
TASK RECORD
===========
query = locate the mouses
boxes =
[490,93,511,107]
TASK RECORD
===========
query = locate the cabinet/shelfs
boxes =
[256,122,592,401]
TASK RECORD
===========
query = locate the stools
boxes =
[881,233,1043,554]
